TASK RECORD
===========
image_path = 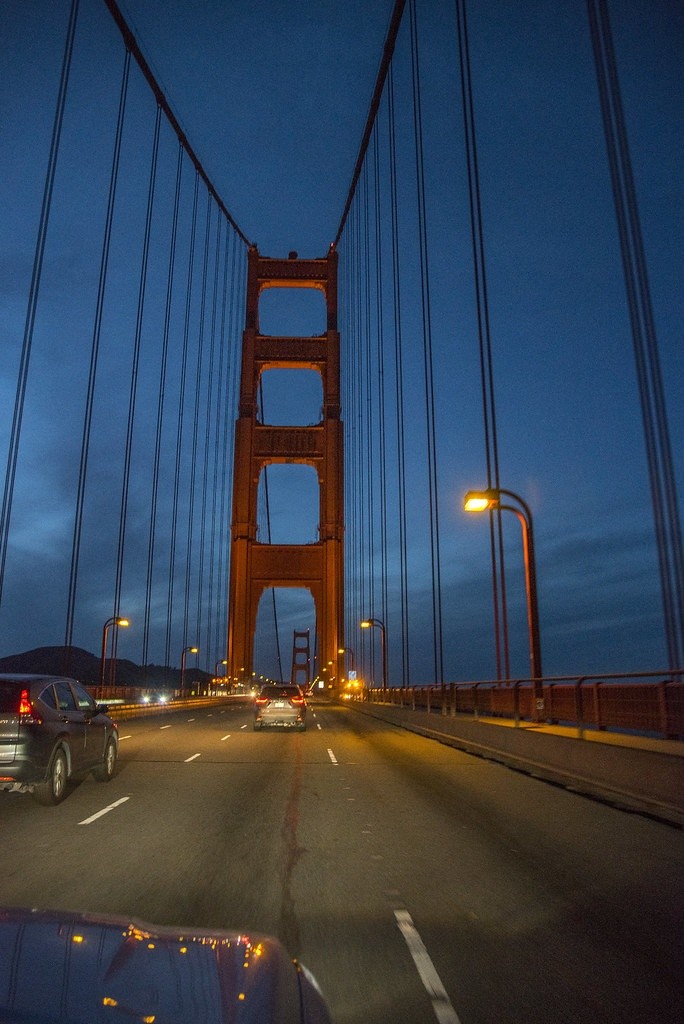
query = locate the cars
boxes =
[0,671,119,807]
[251,683,309,733]
[0,903,335,1024]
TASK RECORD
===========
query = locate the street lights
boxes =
[322,659,341,700]
[99,617,130,701]
[358,617,389,704]
[463,487,548,722]
[234,667,245,695]
[338,646,355,701]
[214,660,228,697]
[179,646,198,698]
